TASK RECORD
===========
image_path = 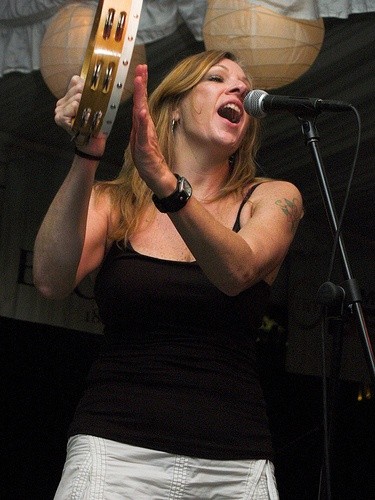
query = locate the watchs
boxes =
[152,172,193,215]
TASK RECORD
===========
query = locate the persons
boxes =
[33,51,306,500]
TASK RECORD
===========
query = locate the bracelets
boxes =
[75,147,103,161]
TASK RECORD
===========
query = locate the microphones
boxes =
[243,90,355,121]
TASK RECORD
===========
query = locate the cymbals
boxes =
[67,0,145,148]
